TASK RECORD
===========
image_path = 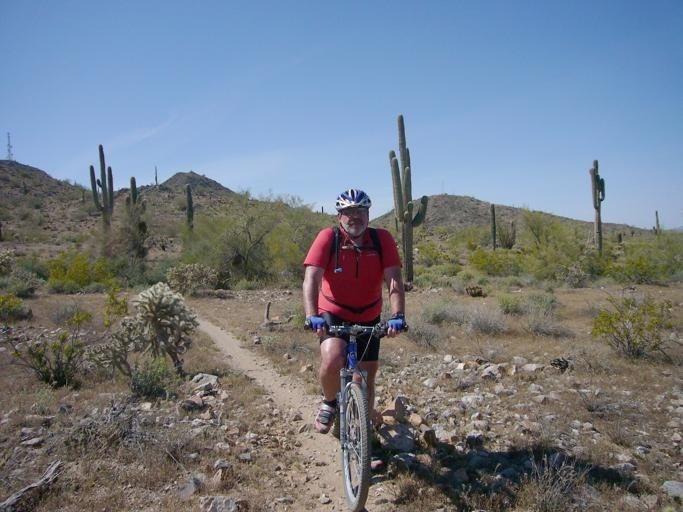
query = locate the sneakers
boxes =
[369,449,385,472]
[314,403,336,435]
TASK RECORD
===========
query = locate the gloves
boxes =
[385,314,407,338]
[305,314,327,336]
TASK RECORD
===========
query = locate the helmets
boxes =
[335,188,371,211]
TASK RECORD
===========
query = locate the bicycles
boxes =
[304,318,409,512]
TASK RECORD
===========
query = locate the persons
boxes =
[302,190,406,434]
